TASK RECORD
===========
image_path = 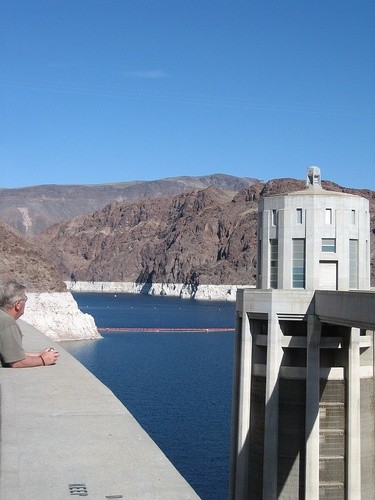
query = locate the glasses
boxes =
[19,296,28,302]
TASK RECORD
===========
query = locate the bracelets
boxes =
[39,355,46,366]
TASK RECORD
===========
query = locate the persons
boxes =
[0,279,60,369]
